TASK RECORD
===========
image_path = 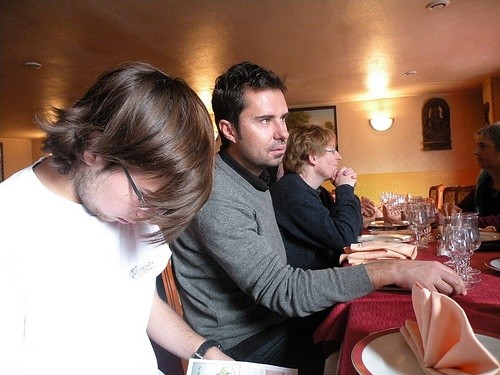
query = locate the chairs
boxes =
[428,184,475,209]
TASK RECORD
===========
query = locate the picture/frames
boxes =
[287,105,338,153]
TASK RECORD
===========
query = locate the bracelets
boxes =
[190,339,224,357]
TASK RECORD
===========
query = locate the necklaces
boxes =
[494,183,499,198]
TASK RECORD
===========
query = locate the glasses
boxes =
[327,147,335,154]
[122,165,159,219]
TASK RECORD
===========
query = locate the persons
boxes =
[169,61,467,369]
[270,125,362,270]
[456,122,500,228]
[0,60,297,375]
[329,189,375,229]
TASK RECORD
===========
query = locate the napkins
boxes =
[339,205,500,375]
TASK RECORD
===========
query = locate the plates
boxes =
[356,233,415,243]
[484,255,500,273]
[369,220,410,227]
[351,325,500,375]
[477,230,500,245]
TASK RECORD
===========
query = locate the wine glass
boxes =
[377,191,482,290]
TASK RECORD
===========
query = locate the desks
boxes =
[333,206,500,375]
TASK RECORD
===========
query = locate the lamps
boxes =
[370,117,395,132]
